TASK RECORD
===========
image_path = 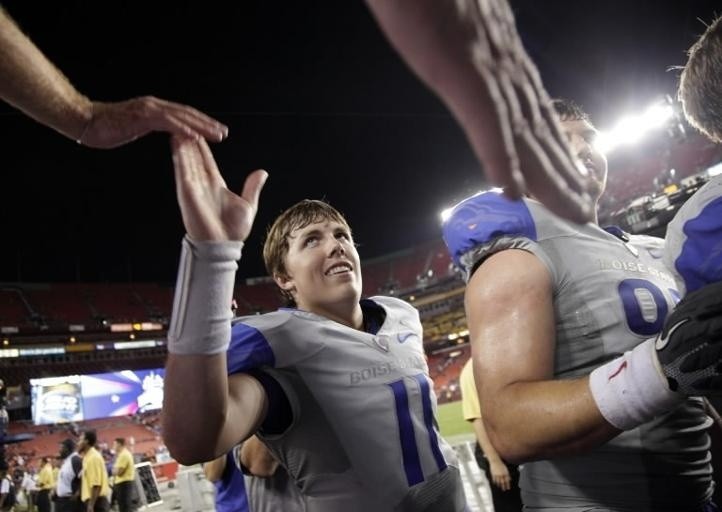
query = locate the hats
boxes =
[57,438,75,449]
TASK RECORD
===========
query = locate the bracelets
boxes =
[587,337,685,429]
[163,233,246,359]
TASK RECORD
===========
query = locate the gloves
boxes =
[587,282,722,431]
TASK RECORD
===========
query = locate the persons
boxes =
[0,429,170,512]
[365,1,596,226]
[231,434,307,511]
[1,6,234,150]
[675,15,722,149]
[0,378,13,427]
[162,481,182,510]
[159,129,474,511]
[440,100,721,512]
[459,356,525,512]
[201,446,249,511]
